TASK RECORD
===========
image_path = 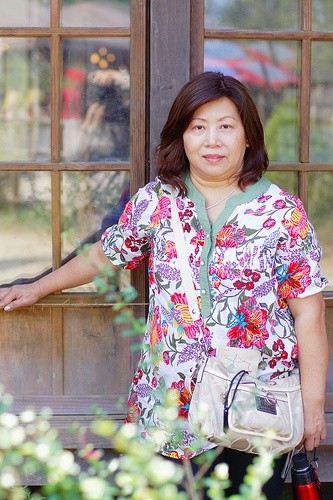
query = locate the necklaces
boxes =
[206,184,239,210]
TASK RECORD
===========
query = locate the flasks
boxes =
[290,453,321,500]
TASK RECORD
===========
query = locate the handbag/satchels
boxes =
[188,346,304,457]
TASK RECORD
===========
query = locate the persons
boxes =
[0,71,328,500]
[0,182,132,288]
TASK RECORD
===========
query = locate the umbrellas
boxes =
[32,3,303,93]
